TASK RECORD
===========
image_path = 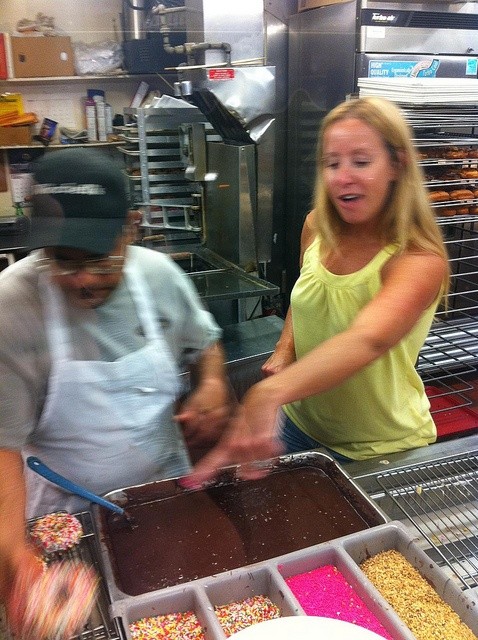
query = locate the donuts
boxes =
[29,511,84,555]
[19,558,102,640]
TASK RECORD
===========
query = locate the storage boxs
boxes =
[124,34,187,74]
[0,125,32,146]
[11,37,75,78]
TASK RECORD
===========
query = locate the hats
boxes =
[20,147,132,253]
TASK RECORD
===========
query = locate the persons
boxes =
[192,96,452,483]
[0,149,230,639]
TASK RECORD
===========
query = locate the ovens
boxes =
[287,0,478,408]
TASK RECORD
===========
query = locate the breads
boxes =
[426,189,451,204]
[437,207,456,217]
[433,169,461,180]
[448,189,474,200]
[457,167,478,180]
[470,205,478,215]
[445,148,466,158]
[455,206,471,214]
[468,150,478,157]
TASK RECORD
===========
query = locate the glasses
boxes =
[31,226,129,276]
